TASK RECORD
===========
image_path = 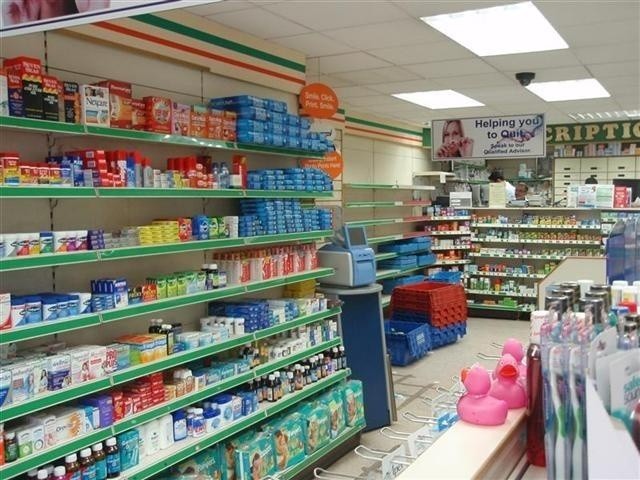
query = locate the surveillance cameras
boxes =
[515,73,536,87]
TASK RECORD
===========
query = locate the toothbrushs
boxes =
[540,304,640,480]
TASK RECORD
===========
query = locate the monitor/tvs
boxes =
[613,179,640,202]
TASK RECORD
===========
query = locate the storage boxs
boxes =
[384,282,467,366]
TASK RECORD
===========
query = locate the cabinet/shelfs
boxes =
[552,157,640,208]
[344,180,639,314]
[0,115,366,480]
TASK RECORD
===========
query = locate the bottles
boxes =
[201,263,219,289]
[64,437,119,480]
[212,162,230,188]
[148,317,173,354]
[105,150,153,187]
[248,346,348,403]
[5,432,17,463]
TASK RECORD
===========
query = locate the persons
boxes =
[494,176,515,202]
[434,120,475,159]
[514,182,530,200]
[8,0,79,25]
